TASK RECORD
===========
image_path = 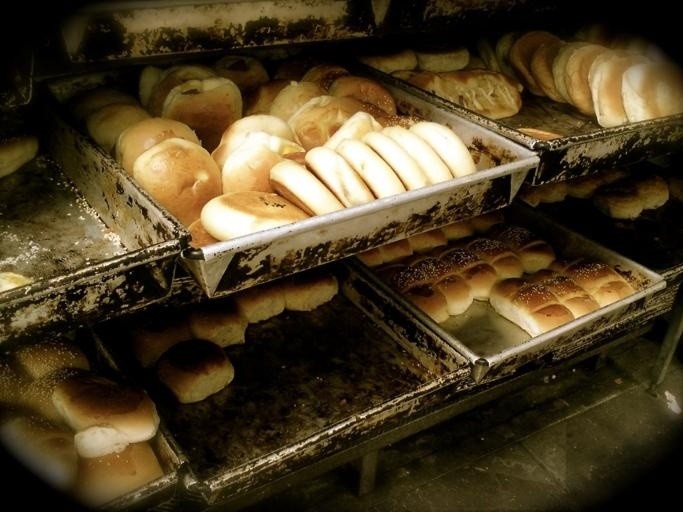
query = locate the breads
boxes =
[83,57,477,252]
[358,211,652,340]
[1,339,166,509]
[2,135,39,178]
[356,23,683,141]
[0,270,34,293]
[518,169,669,220]
[136,260,338,403]
